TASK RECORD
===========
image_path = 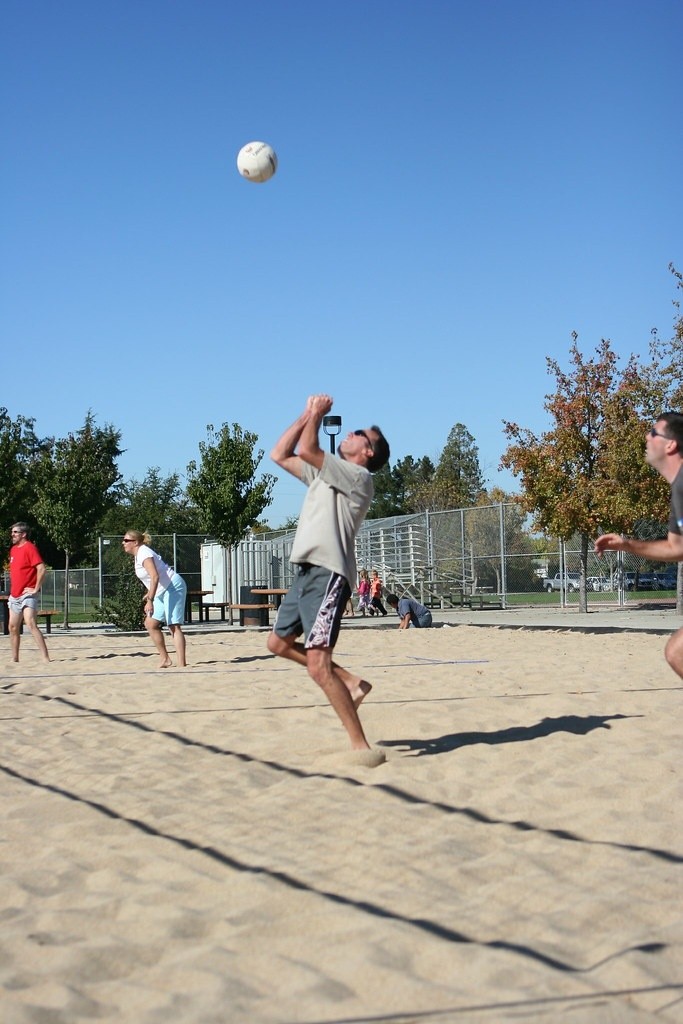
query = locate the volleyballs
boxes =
[236,140,278,183]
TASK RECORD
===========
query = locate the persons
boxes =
[593,413,683,681]
[356,570,379,618]
[266,393,390,750]
[366,570,388,617]
[386,594,453,629]
[122,529,187,669]
[7,521,52,662]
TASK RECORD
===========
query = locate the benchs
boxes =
[227,605,276,626]
[36,609,60,634]
[193,601,229,622]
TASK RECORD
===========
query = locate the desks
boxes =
[0,595,23,635]
[250,589,289,613]
[183,591,214,624]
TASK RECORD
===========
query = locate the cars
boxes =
[623,571,652,591]
[650,572,677,590]
[587,577,608,591]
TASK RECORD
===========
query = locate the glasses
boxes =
[122,538,136,542]
[355,430,374,451]
[651,427,674,440]
[12,531,23,534]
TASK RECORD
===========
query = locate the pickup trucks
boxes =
[543,572,592,592]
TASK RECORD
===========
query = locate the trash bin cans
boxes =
[239,585,270,626]
